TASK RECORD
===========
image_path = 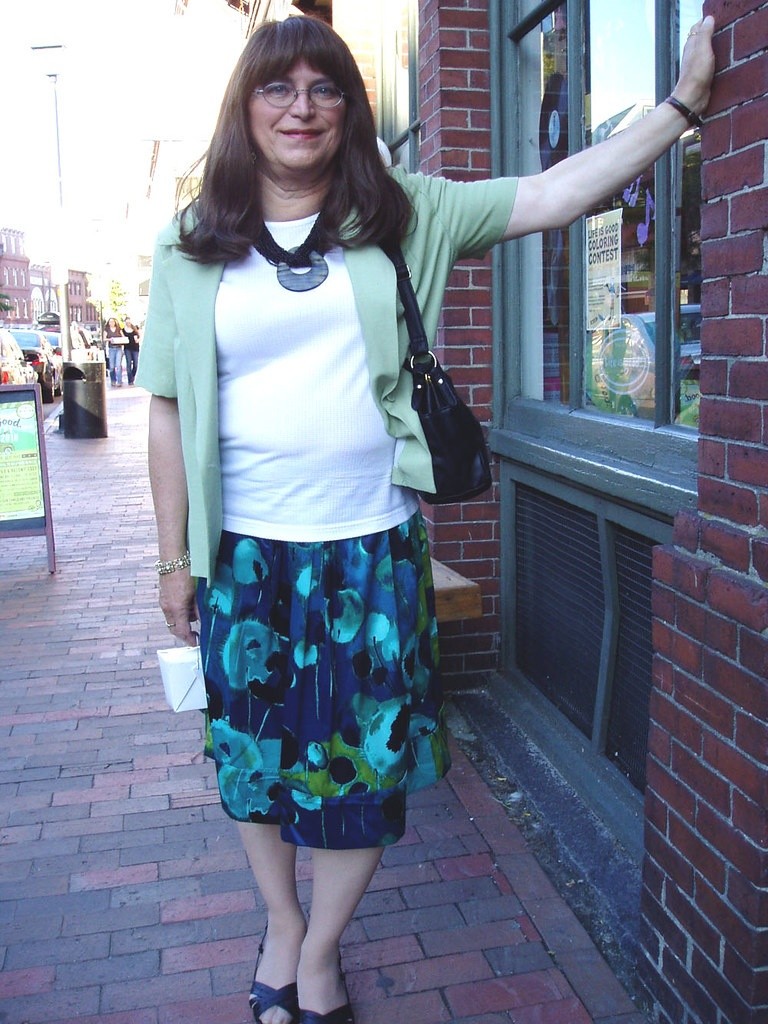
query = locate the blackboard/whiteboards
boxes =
[0,385,53,539]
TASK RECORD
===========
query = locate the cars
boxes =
[584,302,703,380]
[0,329,38,384]
[11,330,63,403]
[43,328,102,382]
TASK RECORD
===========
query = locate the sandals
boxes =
[248,919,354,1024]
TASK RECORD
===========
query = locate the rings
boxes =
[687,30,698,38]
[165,619,176,628]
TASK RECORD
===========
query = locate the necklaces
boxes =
[239,178,351,294]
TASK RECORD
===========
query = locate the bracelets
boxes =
[666,94,704,130]
[150,552,195,579]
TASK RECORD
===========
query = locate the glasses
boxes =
[251,83,346,108]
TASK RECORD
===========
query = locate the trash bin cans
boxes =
[63,361,109,439]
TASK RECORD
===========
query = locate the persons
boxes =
[120,317,139,387]
[102,317,128,388]
[117,7,722,1023]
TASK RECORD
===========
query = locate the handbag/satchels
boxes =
[380,242,493,506]
[134,335,140,343]
